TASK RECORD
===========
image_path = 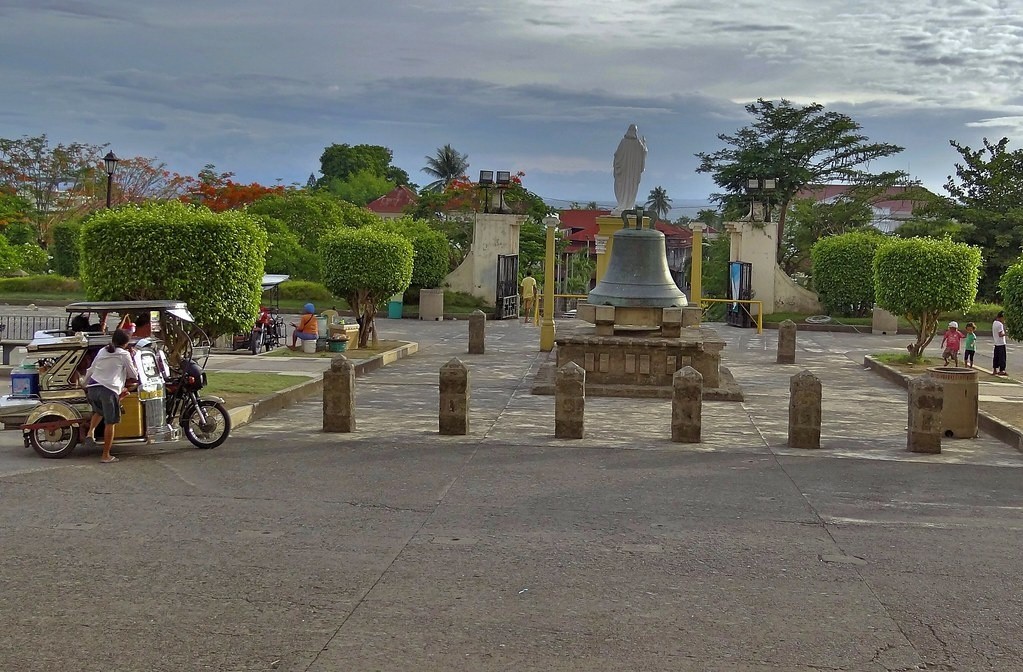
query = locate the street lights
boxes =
[103,150,121,209]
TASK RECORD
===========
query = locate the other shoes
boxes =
[525,318,532,323]
[285,344,295,351]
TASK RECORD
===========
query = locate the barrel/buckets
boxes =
[303,340,316,353]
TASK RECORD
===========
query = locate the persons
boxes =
[286,303,319,350]
[255,304,272,342]
[941,321,966,367]
[613,123,648,209]
[520,270,538,323]
[963,322,977,369]
[991,310,1009,375]
[72,313,157,465]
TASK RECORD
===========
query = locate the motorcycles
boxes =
[229,274,289,355]
[0,299,232,458]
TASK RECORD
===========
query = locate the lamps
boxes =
[746,177,758,193]
[480,171,494,183]
[762,178,776,192]
[497,171,510,184]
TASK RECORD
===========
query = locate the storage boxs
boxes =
[115,394,144,437]
[330,323,360,350]
[10,367,40,397]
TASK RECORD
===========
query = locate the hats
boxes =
[948,322,958,329]
[303,303,314,313]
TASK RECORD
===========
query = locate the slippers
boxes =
[999,372,1008,375]
[85,435,95,447]
[99,456,118,463]
